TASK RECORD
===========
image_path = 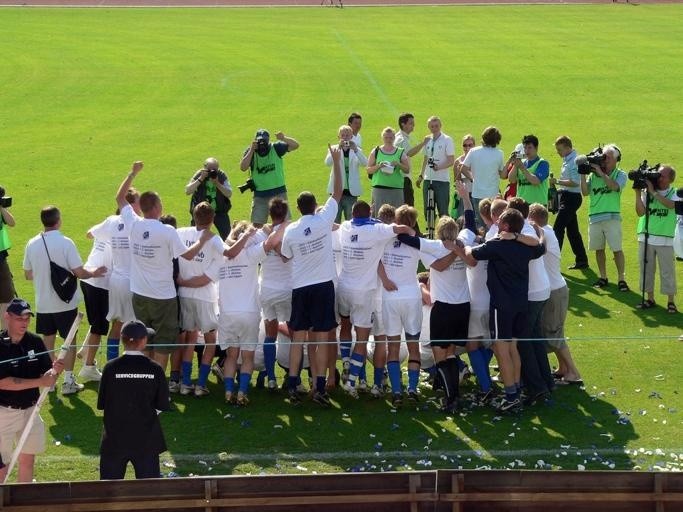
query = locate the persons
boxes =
[90,320,171,478]
[629,163,677,316]
[0,301,65,485]
[671,190,683,343]
[576,141,630,294]
[0,186,18,315]
[22,207,107,394]
[80,110,593,416]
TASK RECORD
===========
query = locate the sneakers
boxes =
[50,361,102,394]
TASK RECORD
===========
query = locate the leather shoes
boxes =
[568,265,587,269]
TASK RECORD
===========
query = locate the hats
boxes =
[256,130,268,141]
[120,320,155,338]
[6,298,34,317]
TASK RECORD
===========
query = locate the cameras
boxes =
[512,150,527,159]
[237,178,256,193]
[254,137,269,156]
[428,157,440,167]
[207,169,217,179]
[343,141,350,146]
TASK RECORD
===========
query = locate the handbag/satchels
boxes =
[49,261,76,303]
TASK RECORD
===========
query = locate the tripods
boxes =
[426,170,436,239]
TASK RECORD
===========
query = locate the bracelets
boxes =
[651,191,657,196]
[599,172,607,177]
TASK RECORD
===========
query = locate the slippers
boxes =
[552,370,583,385]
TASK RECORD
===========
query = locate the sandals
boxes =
[638,300,654,308]
[593,278,607,287]
[668,302,675,310]
[618,281,628,292]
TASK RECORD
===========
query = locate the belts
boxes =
[0,399,36,410]
